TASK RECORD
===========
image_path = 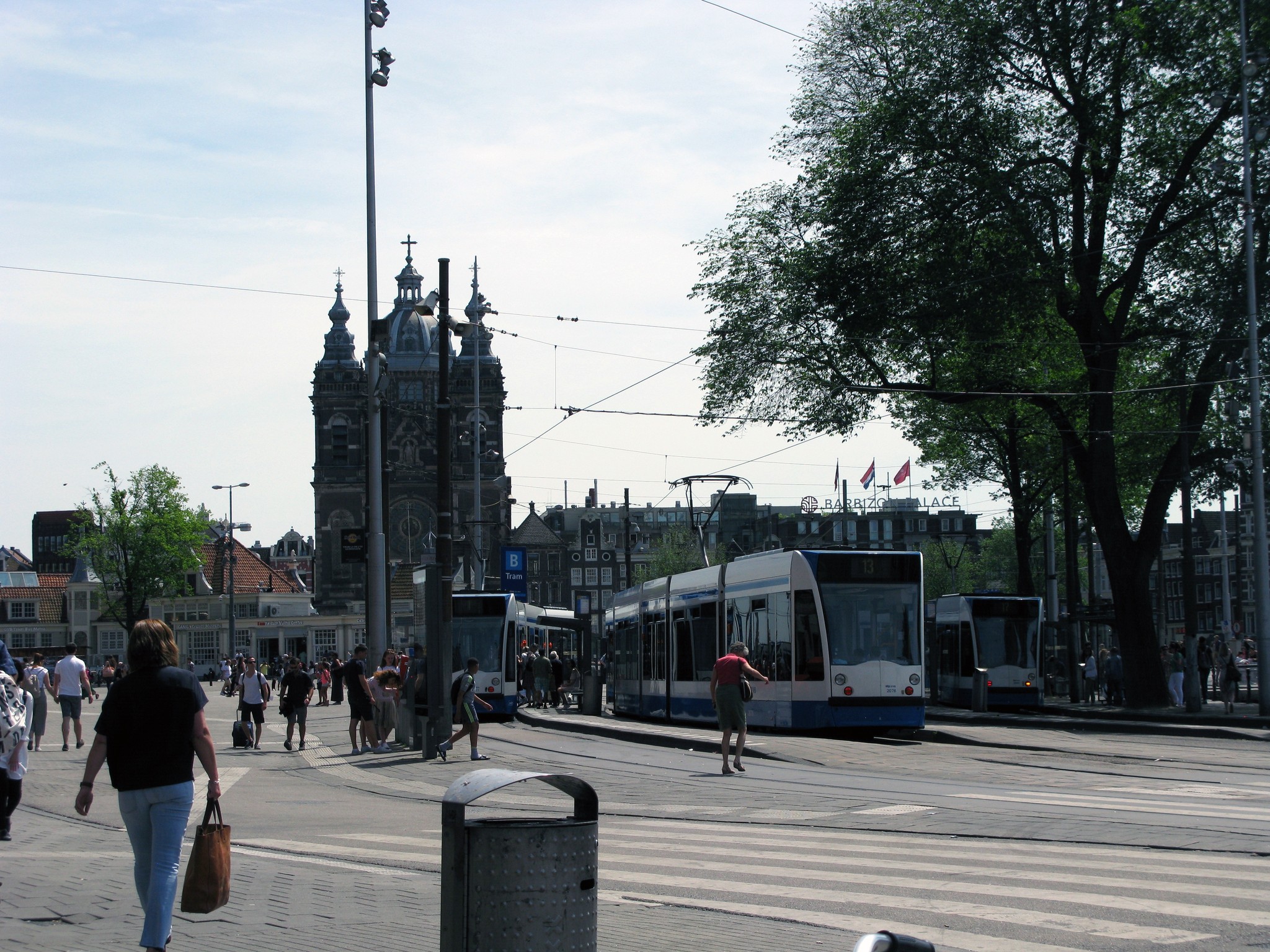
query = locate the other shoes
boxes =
[220,691,239,697]
[76,739,85,749]
[316,700,341,707]
[272,689,277,691]
[522,697,571,709]
[96,694,99,699]
[0,816,12,841]
[352,742,392,755]
[62,744,69,751]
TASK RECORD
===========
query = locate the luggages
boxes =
[232,709,254,748]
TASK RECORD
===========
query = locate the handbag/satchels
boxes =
[181,798,231,913]
[332,663,343,679]
[103,661,115,677]
[88,669,94,678]
[738,656,753,702]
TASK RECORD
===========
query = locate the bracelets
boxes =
[207,777,221,784]
[80,781,94,790]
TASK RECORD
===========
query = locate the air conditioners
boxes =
[267,604,280,617]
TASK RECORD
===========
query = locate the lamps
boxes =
[640,547,644,552]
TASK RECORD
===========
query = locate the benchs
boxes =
[569,691,584,710]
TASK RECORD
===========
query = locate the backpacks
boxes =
[25,668,45,699]
[451,672,474,701]
[243,671,270,702]
[522,652,536,672]
[276,669,282,677]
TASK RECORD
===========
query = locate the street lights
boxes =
[363,0,397,665]
[1209,0,1270,714]
[212,482,252,665]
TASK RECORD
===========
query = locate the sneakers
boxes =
[284,741,293,750]
[28,738,42,751]
[255,744,261,749]
[244,738,254,750]
[299,740,305,750]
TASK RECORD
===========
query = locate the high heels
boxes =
[722,760,746,774]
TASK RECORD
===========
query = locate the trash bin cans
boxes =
[441,768,599,952]
[88,671,97,683]
[397,699,410,746]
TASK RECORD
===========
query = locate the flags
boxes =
[893,457,910,485]
[859,457,875,490]
[834,458,839,492]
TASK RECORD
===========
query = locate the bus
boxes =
[452,559,582,716]
[924,536,1046,710]
[601,475,927,745]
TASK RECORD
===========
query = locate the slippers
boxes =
[471,755,491,761]
[435,745,446,762]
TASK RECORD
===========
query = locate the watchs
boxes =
[307,697,311,701]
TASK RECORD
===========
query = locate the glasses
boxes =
[248,661,256,664]
[290,664,300,669]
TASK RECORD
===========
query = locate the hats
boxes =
[282,654,288,658]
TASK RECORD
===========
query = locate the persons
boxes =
[260,652,345,706]
[515,643,581,710]
[217,650,246,698]
[186,656,195,674]
[279,657,315,751]
[1080,630,1259,719]
[342,642,404,754]
[600,652,607,669]
[203,668,215,681]
[0,631,130,841]
[75,618,222,952]
[238,656,270,750]
[709,642,770,775]
[435,656,493,761]
